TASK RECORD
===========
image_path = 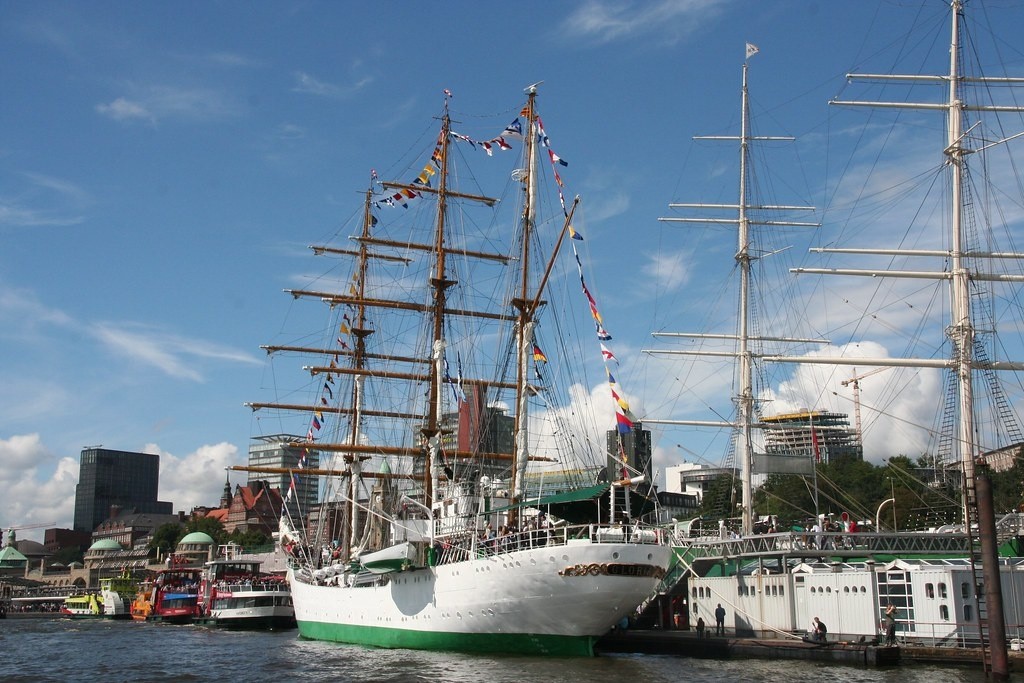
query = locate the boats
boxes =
[58,554,297,629]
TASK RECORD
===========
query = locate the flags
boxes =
[278,101,640,541]
[813,426,821,464]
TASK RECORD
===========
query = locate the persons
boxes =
[696,617,705,637]
[812,617,827,642]
[714,604,727,636]
[433,503,633,563]
[695,512,858,551]
[884,603,900,647]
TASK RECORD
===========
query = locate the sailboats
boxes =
[223,82,679,660]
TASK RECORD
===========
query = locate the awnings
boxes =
[0,572,291,616]
